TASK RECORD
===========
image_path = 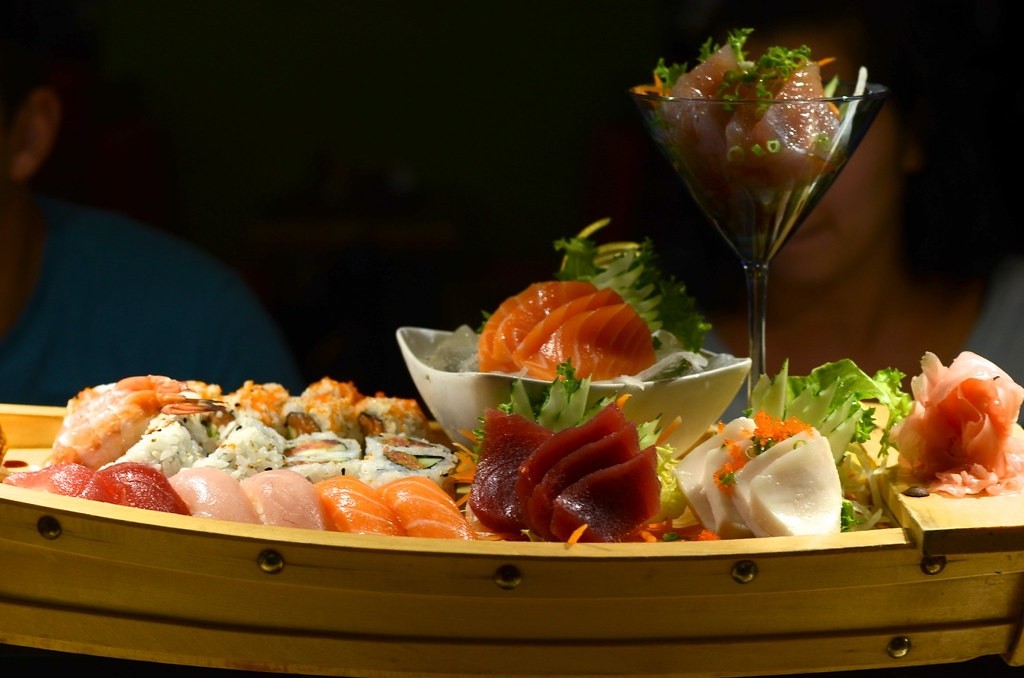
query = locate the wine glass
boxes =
[625,68,890,416]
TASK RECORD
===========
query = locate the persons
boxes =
[579,0,1023,427]
[0,30,304,408]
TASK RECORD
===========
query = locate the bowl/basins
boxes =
[394,319,752,468]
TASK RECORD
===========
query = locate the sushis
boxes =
[53,374,461,496]
